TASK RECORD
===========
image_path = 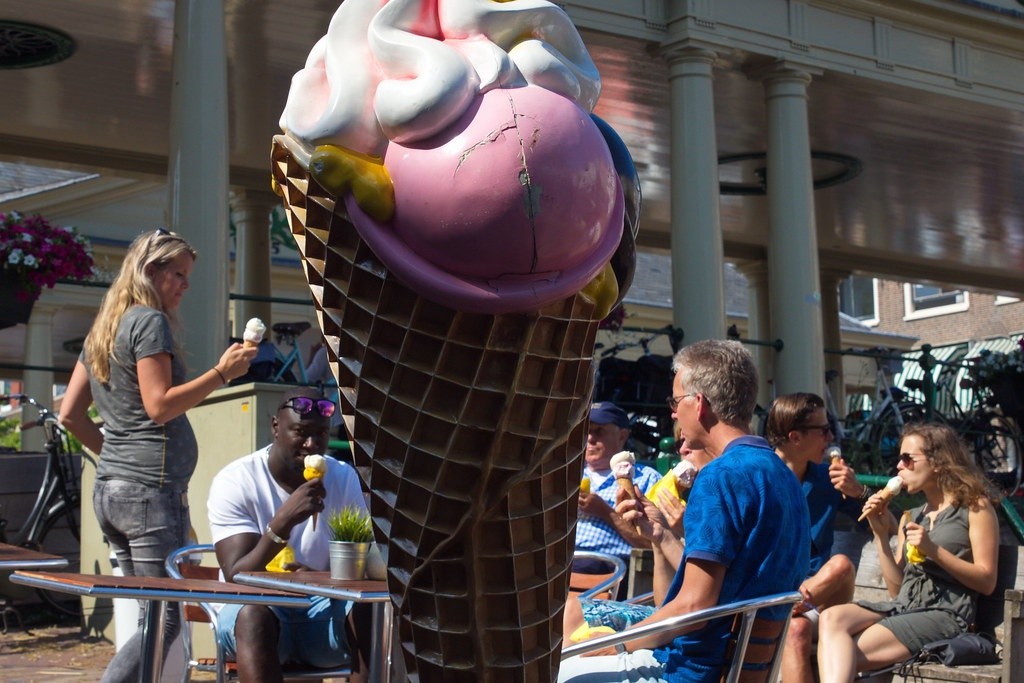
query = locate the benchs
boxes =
[800,534,1024,683]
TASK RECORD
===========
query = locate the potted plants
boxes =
[327,503,376,582]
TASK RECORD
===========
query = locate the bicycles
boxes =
[828,344,1024,500]
[591,324,679,426]
[233,319,340,414]
[0,393,87,621]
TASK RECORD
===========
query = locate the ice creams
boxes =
[610,450,642,537]
[269,0,640,683]
[827,446,846,499]
[304,454,327,532]
[858,476,903,522]
[226,317,266,383]
[673,459,698,492]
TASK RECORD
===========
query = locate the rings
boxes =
[618,515,623,520]
[318,500,321,504]
[879,512,883,515]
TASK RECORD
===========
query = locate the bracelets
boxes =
[212,367,225,384]
[266,521,288,543]
[859,484,868,498]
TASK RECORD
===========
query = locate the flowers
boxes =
[959,338,1024,388]
[0,208,95,301]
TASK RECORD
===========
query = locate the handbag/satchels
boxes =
[898,632,1003,676]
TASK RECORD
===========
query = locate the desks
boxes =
[0,538,392,683]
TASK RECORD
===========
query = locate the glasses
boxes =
[896,453,928,467]
[149,227,170,245]
[666,393,711,413]
[785,424,830,439]
[673,438,685,455]
[276,397,337,418]
[588,428,621,438]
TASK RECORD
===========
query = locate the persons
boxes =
[815,421,998,683]
[562,413,764,654]
[207,386,411,683]
[305,332,354,463]
[569,401,666,602]
[555,336,812,683]
[57,227,258,683]
[760,390,898,683]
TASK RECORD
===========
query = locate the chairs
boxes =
[559,549,801,683]
[168,544,352,683]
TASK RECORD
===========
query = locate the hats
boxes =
[590,402,629,429]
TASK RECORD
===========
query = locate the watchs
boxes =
[614,630,626,653]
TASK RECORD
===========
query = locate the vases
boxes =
[989,373,1024,417]
[0,259,52,329]
